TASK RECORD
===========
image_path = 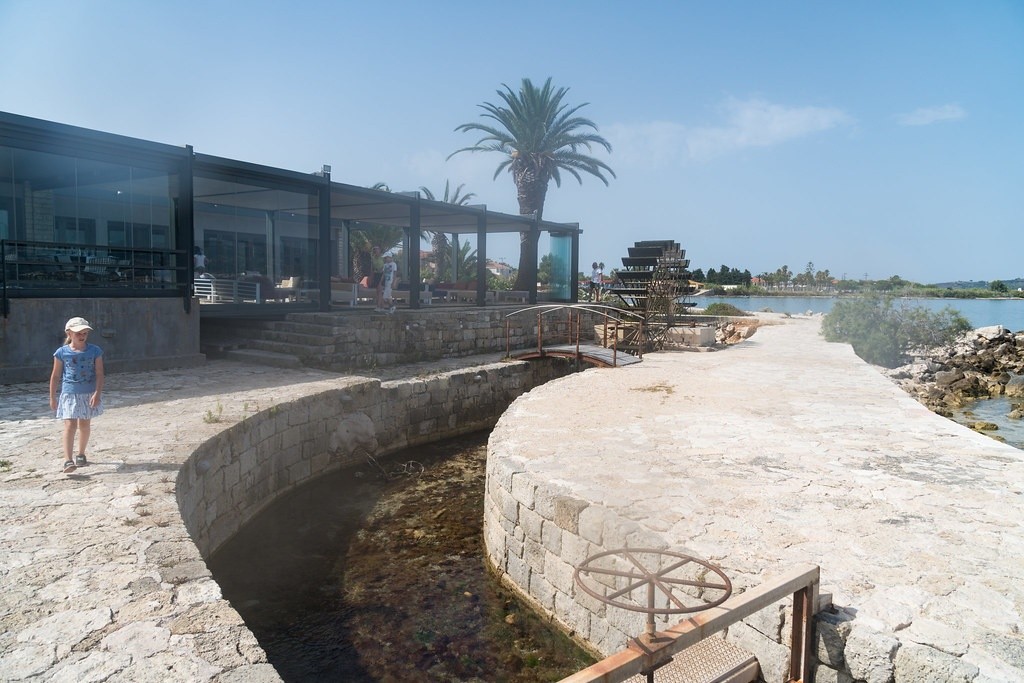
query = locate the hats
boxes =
[65,317,94,332]
[381,252,393,258]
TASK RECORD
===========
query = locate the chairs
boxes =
[36,248,131,287]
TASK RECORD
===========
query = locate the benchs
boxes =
[274,280,529,303]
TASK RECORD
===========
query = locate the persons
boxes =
[193,248,208,276]
[371,244,399,315]
[588,262,605,302]
[50,316,105,474]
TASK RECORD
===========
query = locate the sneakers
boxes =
[62,460,77,473]
[385,307,396,314]
[76,454,87,466]
[374,307,385,312]
